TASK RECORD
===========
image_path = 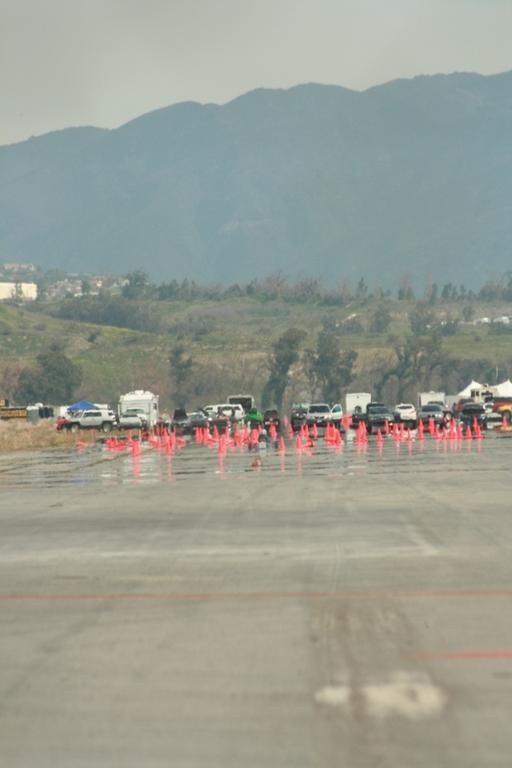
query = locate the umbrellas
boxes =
[67,399,96,418]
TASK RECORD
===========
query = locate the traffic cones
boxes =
[123,456,306,478]
[76,436,86,451]
